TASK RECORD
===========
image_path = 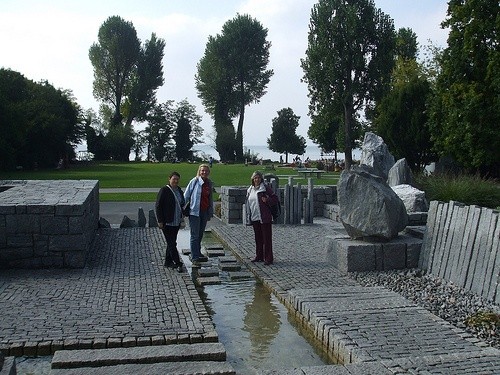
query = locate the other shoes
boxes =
[177,262,183,273]
[192,255,208,262]
[264,259,273,265]
[164,262,178,268]
[251,257,264,262]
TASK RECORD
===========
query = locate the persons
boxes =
[279,155,345,172]
[155,172,186,273]
[246,172,280,265]
[184,164,214,262]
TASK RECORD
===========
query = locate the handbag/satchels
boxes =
[263,191,281,217]
[182,200,190,217]
[179,218,186,229]
